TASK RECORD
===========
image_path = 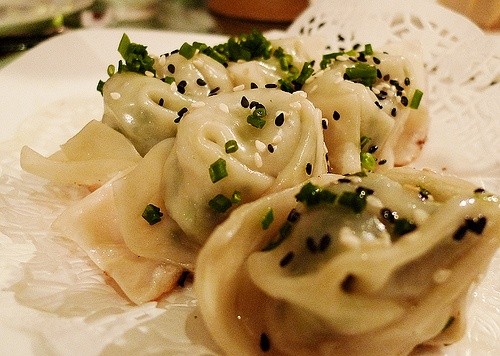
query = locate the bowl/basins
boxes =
[206,0,309,35]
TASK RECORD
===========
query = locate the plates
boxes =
[0,28,499,356]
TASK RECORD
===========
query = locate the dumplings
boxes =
[19,37,499,354]
